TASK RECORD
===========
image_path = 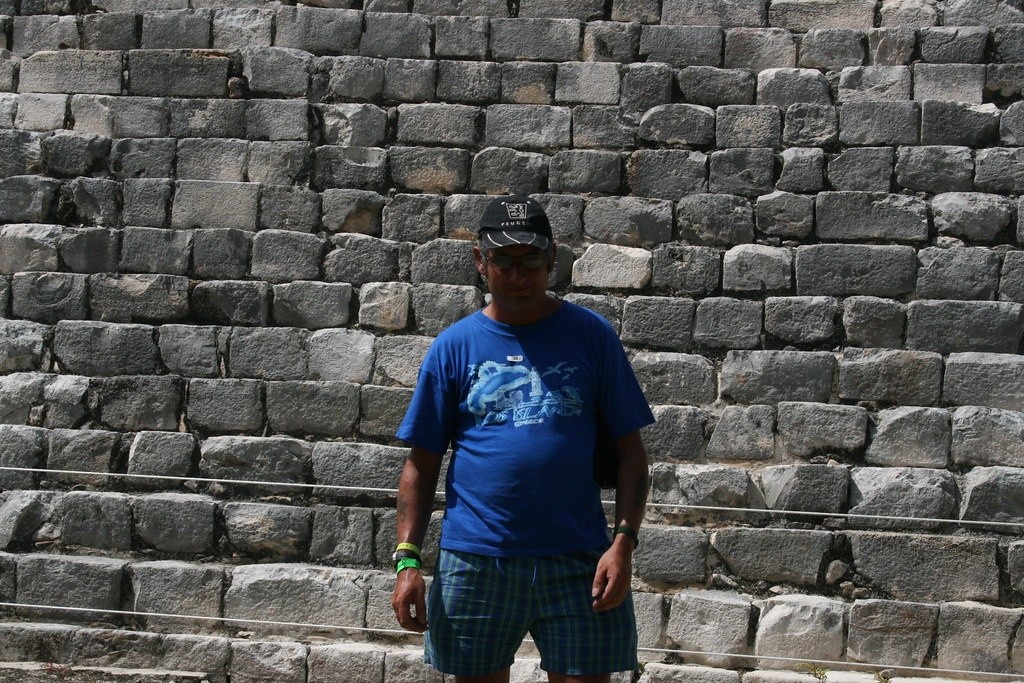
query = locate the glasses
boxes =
[482,248,551,270]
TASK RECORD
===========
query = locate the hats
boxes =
[476,193,553,249]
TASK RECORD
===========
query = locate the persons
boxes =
[391,195,656,683]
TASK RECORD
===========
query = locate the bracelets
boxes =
[396,543,422,557]
[397,558,420,575]
[613,526,639,549]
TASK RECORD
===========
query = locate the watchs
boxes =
[392,550,423,569]
